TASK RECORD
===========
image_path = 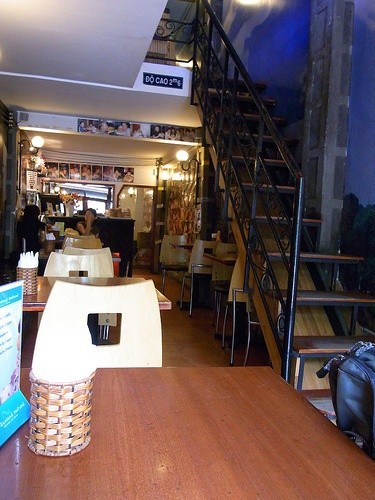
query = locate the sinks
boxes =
[96,214,105,216]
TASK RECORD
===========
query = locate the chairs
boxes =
[62,246,114,278]
[159,235,260,364]
[30,279,163,368]
[37,251,118,346]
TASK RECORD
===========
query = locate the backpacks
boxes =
[316,340,375,460]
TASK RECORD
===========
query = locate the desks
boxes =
[37,248,121,262]
[203,252,248,349]
[0,366,375,500]
[170,243,212,310]
[0,275,173,345]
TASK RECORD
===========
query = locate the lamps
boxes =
[176,150,202,208]
[16,135,45,195]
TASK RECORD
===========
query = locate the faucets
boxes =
[96,209,98,210]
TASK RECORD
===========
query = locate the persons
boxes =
[46,165,134,183]
[79,122,196,142]
[6,204,47,270]
[75,208,107,249]
